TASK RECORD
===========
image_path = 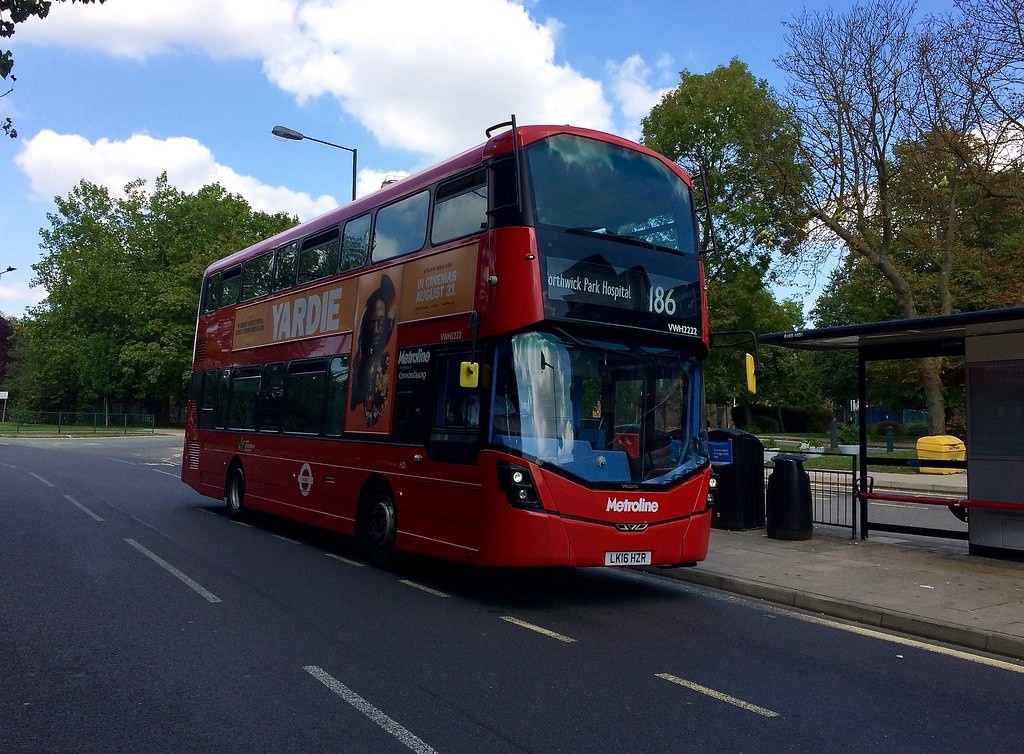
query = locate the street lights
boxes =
[271,126,357,200]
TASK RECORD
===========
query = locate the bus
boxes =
[181,115,762,591]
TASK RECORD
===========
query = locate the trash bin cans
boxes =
[707,427,766,532]
[916,435,966,475]
[767,456,813,541]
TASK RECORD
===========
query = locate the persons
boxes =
[441,356,521,438]
[350,274,392,424]
